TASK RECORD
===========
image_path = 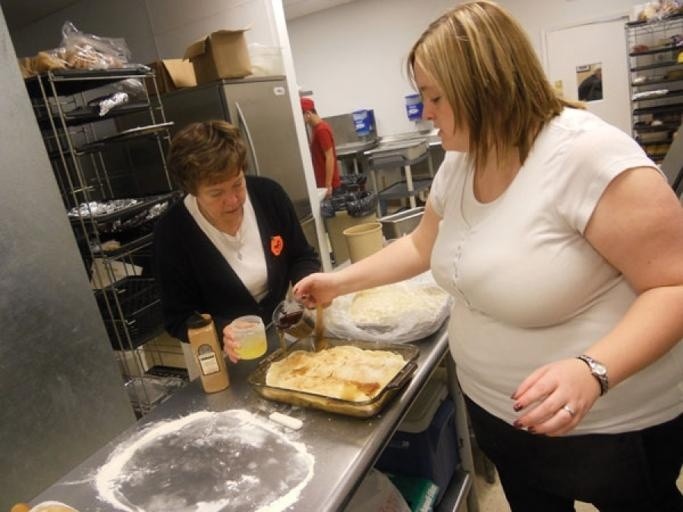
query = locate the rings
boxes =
[563,406,574,418]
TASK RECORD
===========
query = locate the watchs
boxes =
[576,354,609,396]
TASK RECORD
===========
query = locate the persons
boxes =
[292,0,683,512]
[300,95,343,265]
[151,119,323,366]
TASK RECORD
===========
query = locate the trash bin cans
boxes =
[323,189,382,265]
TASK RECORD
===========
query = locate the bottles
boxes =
[187,314,230,394]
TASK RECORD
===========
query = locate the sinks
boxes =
[364,139,428,159]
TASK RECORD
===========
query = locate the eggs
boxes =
[9,502,31,512]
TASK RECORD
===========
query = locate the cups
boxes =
[226,315,268,361]
[269,295,316,341]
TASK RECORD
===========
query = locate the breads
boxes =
[18,42,124,79]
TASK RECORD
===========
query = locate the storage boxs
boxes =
[140,57,198,94]
[183,26,253,84]
[372,367,459,509]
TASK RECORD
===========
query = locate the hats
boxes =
[301,99,314,113]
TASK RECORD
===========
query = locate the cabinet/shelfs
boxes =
[623,13,682,166]
[25,58,187,420]
